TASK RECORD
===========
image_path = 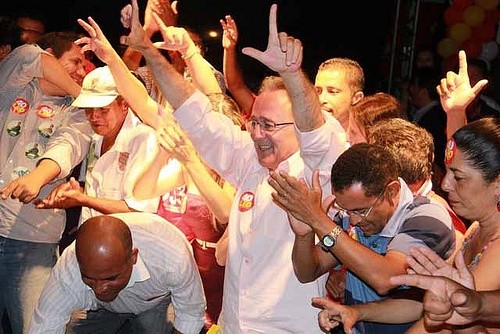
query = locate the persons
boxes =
[0,0,500,334]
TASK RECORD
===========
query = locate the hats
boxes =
[70,65,120,108]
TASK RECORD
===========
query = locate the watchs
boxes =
[320,226,344,251]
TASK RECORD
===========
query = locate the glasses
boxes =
[246,117,294,131]
[332,188,386,219]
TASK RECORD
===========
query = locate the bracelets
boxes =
[181,48,198,59]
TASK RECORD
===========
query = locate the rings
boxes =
[281,191,289,196]
